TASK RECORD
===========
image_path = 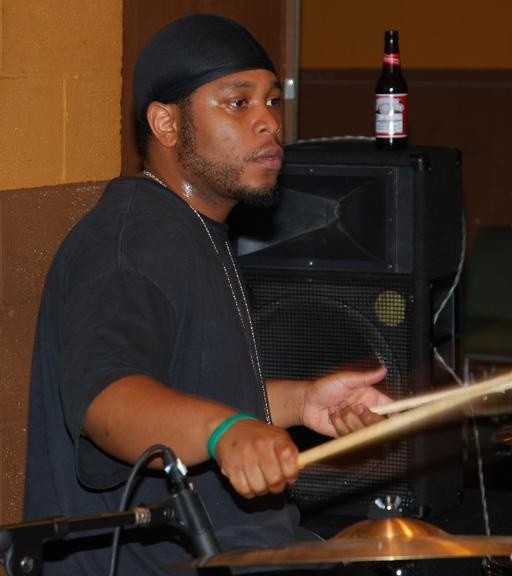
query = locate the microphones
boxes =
[161,446,231,573]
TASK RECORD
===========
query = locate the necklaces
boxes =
[142,171,272,426]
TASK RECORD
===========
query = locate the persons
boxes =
[23,13,400,576]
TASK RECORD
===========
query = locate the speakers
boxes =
[221,141,465,546]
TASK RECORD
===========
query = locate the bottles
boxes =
[374,22,409,148]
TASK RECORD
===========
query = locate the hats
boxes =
[133,15,275,138]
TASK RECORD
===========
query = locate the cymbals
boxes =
[179,515,510,570]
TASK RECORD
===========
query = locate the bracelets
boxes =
[207,412,256,458]
